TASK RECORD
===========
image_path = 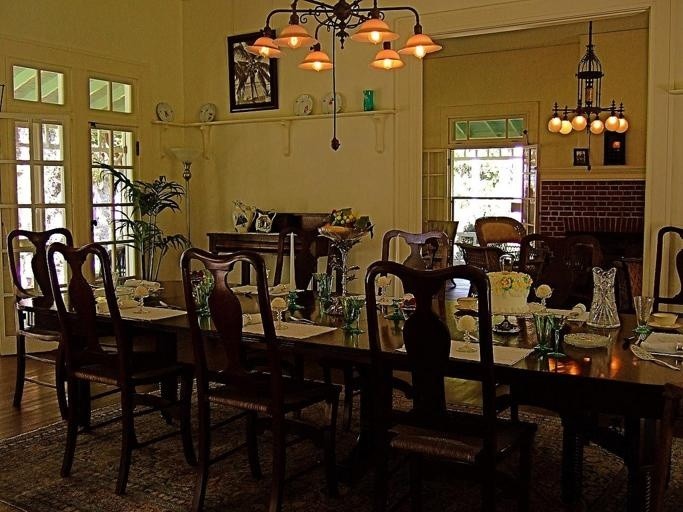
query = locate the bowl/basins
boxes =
[652,313,678,327]
[458,297,478,308]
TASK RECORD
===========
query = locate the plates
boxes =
[454,304,478,313]
[157,102,175,122]
[564,331,611,349]
[647,322,682,331]
[199,103,216,123]
[293,92,342,116]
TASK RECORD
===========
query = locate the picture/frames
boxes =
[227,29,279,113]
[605,130,626,166]
[572,148,589,167]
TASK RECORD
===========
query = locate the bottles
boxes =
[363,90,374,111]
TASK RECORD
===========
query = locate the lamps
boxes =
[243,0,443,151]
[547,19,628,170]
[172,147,204,247]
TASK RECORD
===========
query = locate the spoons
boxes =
[631,344,680,370]
[289,315,316,324]
[159,301,183,309]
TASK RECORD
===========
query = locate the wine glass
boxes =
[269,294,289,331]
[452,314,480,352]
[533,287,554,305]
[376,276,394,302]
[532,312,569,359]
[633,297,654,332]
[135,283,150,313]
[312,273,366,335]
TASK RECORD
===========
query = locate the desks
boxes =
[208,233,332,286]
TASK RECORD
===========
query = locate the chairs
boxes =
[650,226,683,489]
[458,244,511,301]
[46,243,195,496]
[175,247,342,511]
[7,228,73,419]
[474,219,526,248]
[341,229,452,432]
[348,261,538,511]
[518,235,633,500]
